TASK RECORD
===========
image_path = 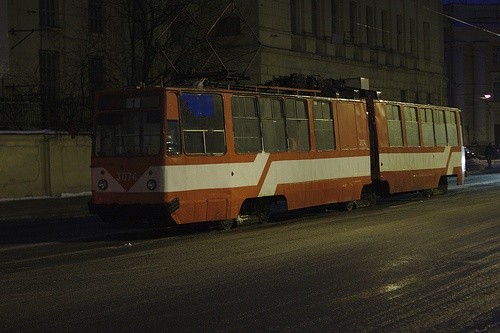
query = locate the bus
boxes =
[89,83,467,232]
